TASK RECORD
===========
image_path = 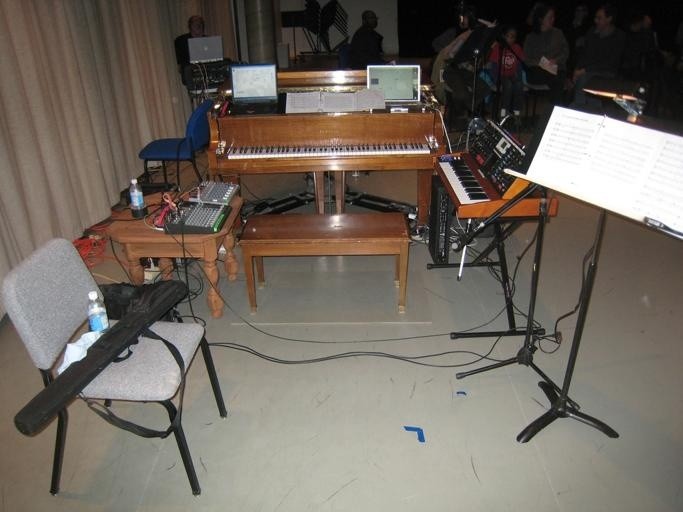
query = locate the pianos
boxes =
[437,152,500,204]
[207,91,448,234]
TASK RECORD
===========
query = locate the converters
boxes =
[253,200,269,213]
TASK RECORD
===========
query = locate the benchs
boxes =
[236,212,413,318]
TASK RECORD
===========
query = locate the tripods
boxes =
[516,209,621,445]
[453,186,582,413]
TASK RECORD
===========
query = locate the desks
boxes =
[109,190,246,316]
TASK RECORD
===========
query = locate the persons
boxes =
[175,14,232,103]
[433,1,683,120]
[344,9,395,68]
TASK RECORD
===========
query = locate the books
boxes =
[284,88,383,114]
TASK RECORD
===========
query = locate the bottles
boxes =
[129,180,143,209]
[86,291,108,334]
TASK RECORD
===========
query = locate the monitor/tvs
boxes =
[187,35,224,64]
[229,63,278,114]
[366,64,421,113]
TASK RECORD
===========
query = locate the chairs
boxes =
[3,236,226,495]
[139,99,214,187]
